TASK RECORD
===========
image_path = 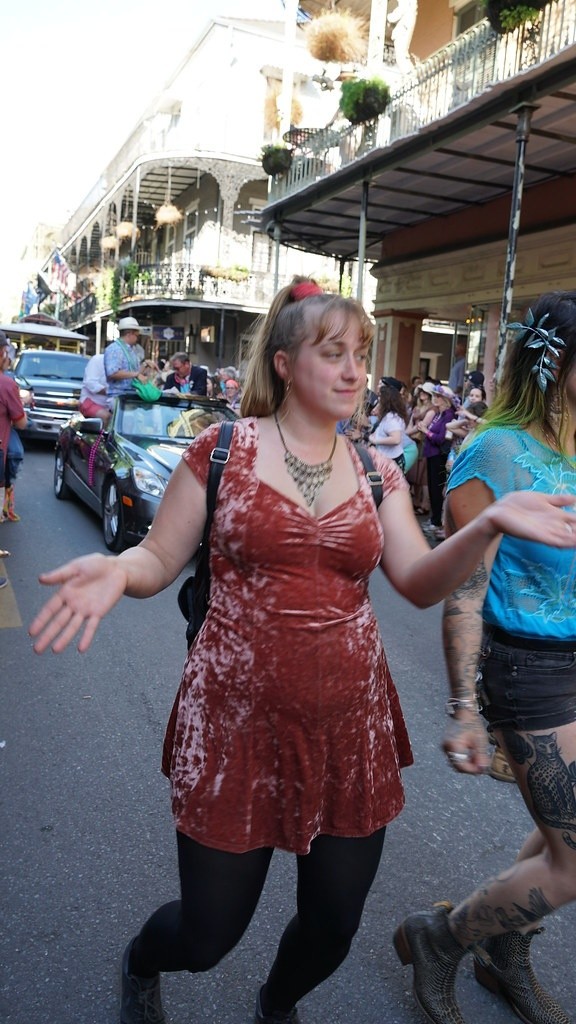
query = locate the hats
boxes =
[418,381,435,396]
[116,317,142,331]
[433,386,454,400]
[0,330,9,346]
[381,377,403,393]
[468,371,484,385]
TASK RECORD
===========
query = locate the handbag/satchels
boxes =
[132,376,162,402]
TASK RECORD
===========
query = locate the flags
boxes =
[17,274,53,318]
[52,250,77,301]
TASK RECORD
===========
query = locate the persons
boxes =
[79,317,246,431]
[0,329,33,589]
[28,277,576,1024]
[393,291,576,1024]
[336,354,490,541]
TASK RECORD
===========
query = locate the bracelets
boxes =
[444,697,477,716]
[474,417,479,422]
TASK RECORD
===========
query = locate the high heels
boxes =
[393,898,465,1024]
[471,923,570,1024]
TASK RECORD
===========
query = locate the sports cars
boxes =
[51,394,241,551]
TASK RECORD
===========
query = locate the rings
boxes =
[446,749,471,762]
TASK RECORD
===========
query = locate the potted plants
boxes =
[339,77,391,124]
[478,0,553,44]
[110,258,139,315]
[260,145,293,175]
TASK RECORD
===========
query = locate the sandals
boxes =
[415,505,429,515]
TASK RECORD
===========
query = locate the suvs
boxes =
[9,349,91,442]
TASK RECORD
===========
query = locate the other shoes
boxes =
[421,517,432,526]
[120,937,167,1023]
[488,749,517,783]
[0,576,8,588]
[423,525,441,534]
[435,529,447,539]
[255,983,300,1024]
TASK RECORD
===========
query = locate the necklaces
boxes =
[536,419,576,471]
[275,413,337,507]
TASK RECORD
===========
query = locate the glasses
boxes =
[172,361,186,371]
